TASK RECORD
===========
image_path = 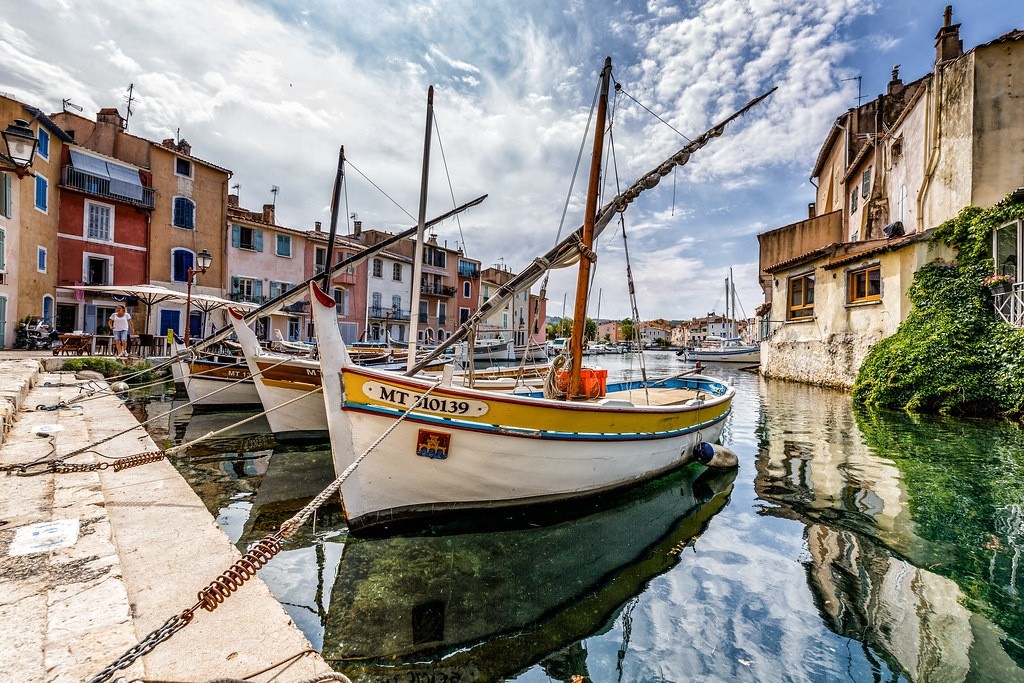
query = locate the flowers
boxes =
[983,274,1015,287]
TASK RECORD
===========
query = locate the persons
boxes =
[109,305,133,357]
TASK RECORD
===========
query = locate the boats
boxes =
[318,445,739,680]
[223,325,629,371]
[166,145,457,407]
[307,56,736,524]
[226,79,548,436]
[170,408,268,463]
[237,438,346,550]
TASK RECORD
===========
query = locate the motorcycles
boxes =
[25,317,59,351]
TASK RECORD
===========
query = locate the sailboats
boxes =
[682,267,760,363]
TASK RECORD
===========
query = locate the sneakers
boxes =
[118,350,128,357]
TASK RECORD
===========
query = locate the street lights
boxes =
[385,304,397,343]
[182,248,213,346]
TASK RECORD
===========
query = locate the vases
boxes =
[989,283,1013,295]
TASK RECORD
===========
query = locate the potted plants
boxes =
[234,276,239,288]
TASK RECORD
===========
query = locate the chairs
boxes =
[95,340,109,355]
[138,334,157,356]
[204,342,220,354]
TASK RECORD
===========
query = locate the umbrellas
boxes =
[52,282,204,335]
[223,294,296,340]
[180,290,256,340]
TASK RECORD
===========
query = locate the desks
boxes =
[58,335,90,356]
[130,335,142,356]
[93,334,115,356]
[153,336,186,356]
